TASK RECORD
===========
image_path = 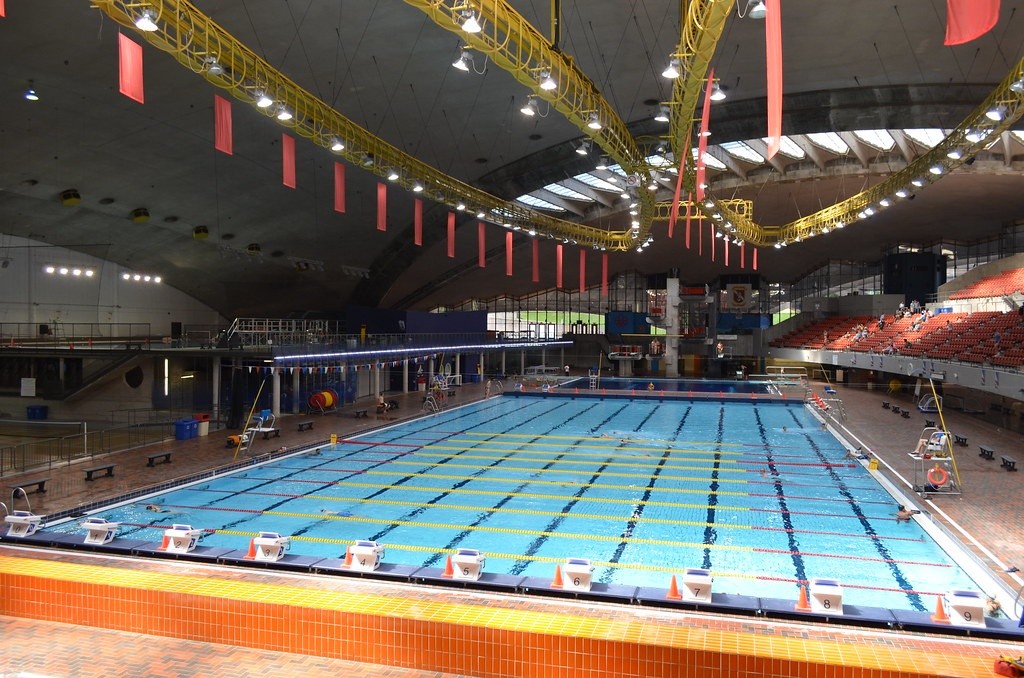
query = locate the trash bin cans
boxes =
[26,405,48,420]
[417,377,426,391]
[192,413,210,436]
[175,418,199,440]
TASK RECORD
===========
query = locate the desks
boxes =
[353,408,369,418]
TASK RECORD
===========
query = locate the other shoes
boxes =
[1006,566,1019,572]
[911,451,919,454]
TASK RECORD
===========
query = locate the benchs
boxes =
[8,478,52,498]
[978,445,995,459]
[259,428,280,440]
[294,420,314,432]
[1000,455,1018,471]
[882,401,952,439]
[389,390,456,410]
[954,433,968,446]
[81,463,119,481]
[145,451,173,468]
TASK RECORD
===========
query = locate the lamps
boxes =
[25,0,1024,252]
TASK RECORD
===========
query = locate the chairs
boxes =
[948,267,1024,300]
[769,310,1024,371]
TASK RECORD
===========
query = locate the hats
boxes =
[898,505,904,511]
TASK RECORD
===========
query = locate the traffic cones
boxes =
[243,538,256,560]
[156,535,169,551]
[550,564,563,588]
[719,390,723,397]
[751,391,755,398]
[441,555,453,579]
[602,388,606,393]
[574,387,579,392]
[811,391,820,404]
[687,390,693,397]
[665,575,682,599]
[341,547,352,567]
[822,402,827,411]
[88,338,93,346]
[930,595,952,623]
[795,585,812,611]
[631,389,634,394]
[660,389,664,396]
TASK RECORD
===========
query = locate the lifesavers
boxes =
[926,467,947,485]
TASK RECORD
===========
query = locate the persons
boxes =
[311,448,321,454]
[846,449,856,457]
[904,339,912,351]
[379,392,387,414]
[1017,302,1024,326]
[823,329,831,346]
[882,342,898,355]
[783,426,787,432]
[945,320,953,330]
[898,504,914,516]
[760,469,767,477]
[876,312,892,333]
[563,364,570,377]
[820,423,827,430]
[993,328,1001,356]
[717,341,723,356]
[894,299,934,332]
[146,505,169,513]
[911,424,946,458]
[843,323,870,342]
